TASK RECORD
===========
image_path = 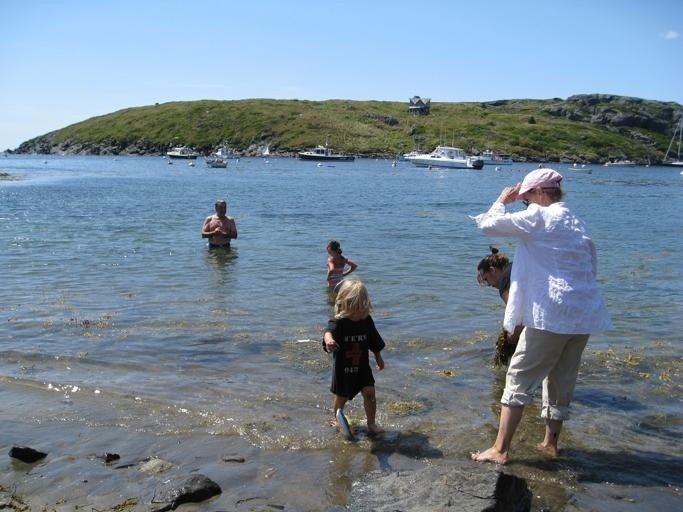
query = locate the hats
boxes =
[511,166,565,202]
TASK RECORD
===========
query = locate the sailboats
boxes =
[660,115,683,167]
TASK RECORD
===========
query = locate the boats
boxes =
[604,160,636,168]
[215,148,240,160]
[403,124,514,170]
[568,167,592,174]
[206,160,215,164]
[296,145,355,161]
[210,160,227,168]
[166,147,199,160]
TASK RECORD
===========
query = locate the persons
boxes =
[202,200,237,249]
[471,168,597,465]
[326,240,357,289]
[322,280,385,433]
[477,248,525,366]
